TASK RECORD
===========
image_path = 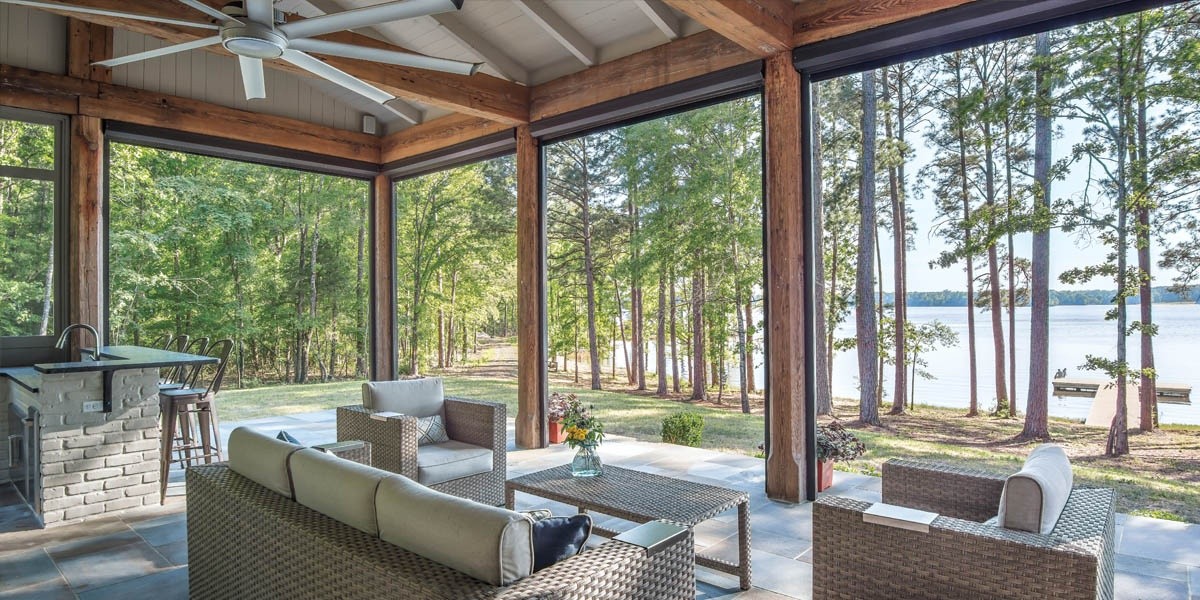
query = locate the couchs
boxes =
[186,425,697,600]
[336,377,507,508]
[813,444,1114,600]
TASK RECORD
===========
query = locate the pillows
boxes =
[275,430,338,458]
[522,508,593,573]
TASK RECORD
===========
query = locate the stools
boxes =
[150,333,234,505]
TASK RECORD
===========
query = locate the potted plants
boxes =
[816,421,866,491]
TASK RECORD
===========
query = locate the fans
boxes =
[8,0,487,105]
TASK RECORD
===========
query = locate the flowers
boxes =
[549,390,605,472]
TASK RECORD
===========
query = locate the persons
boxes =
[1054,369,1063,378]
[1062,368,1067,377]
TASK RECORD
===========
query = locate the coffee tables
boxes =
[505,461,752,590]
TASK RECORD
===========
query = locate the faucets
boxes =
[54,324,101,361]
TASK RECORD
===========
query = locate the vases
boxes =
[550,417,568,443]
[572,445,604,477]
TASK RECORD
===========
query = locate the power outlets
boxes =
[83,400,104,413]
